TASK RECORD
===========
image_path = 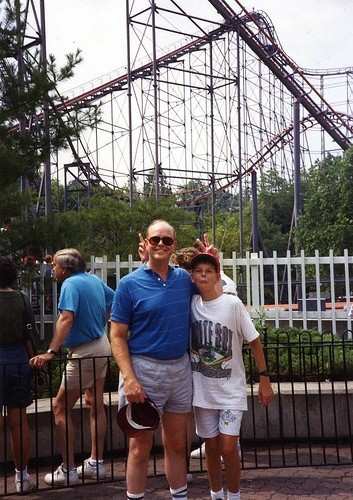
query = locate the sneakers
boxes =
[191,444,208,458]
[14,475,34,492]
[76,459,106,480]
[44,462,78,487]
[220,449,241,464]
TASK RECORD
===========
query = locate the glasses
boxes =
[147,236,175,247]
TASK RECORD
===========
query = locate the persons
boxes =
[171,248,242,461]
[23,254,55,314]
[29,249,115,484]
[138,233,273,500]
[0,257,38,491]
[111,219,222,500]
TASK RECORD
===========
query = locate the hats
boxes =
[191,253,220,276]
[116,396,161,438]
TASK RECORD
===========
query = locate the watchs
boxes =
[47,349,57,356]
[260,370,269,377]
